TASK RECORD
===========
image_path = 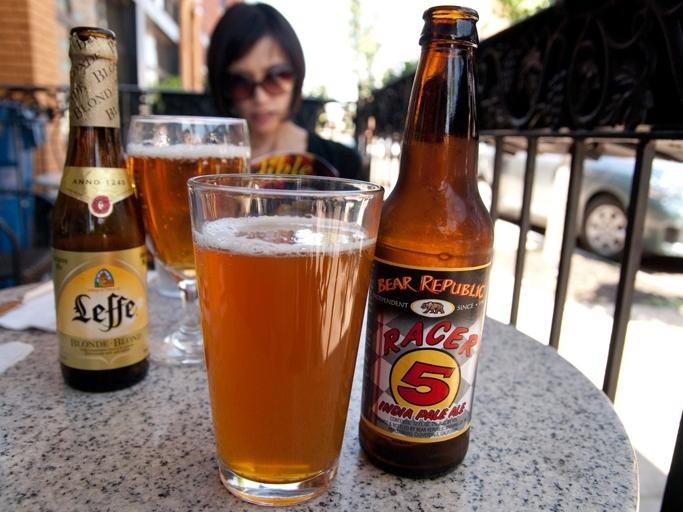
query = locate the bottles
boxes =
[358,6,494,476]
[51,27,149,393]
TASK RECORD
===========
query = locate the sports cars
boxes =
[478,142,683,260]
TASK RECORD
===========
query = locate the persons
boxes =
[199,0,363,182]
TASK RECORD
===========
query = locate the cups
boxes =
[187,173,384,509]
[125,115,253,367]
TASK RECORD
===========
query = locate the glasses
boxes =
[222,63,296,101]
